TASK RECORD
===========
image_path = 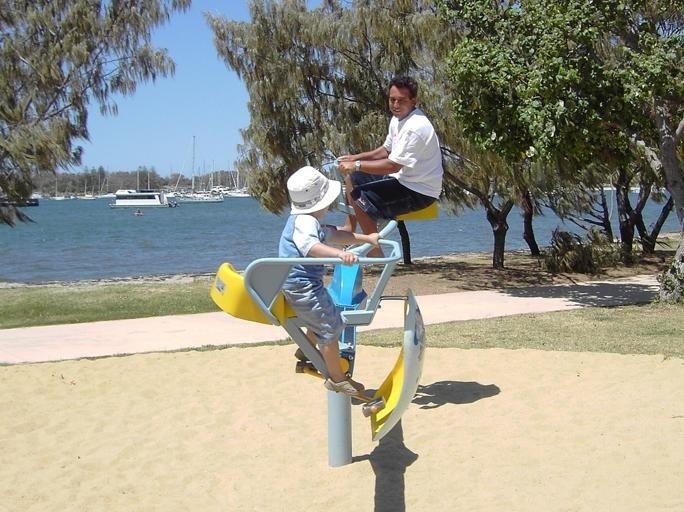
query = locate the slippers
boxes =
[294,348,310,362]
[324,376,365,396]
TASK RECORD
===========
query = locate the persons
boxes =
[339,76,440,256]
[278,165,383,396]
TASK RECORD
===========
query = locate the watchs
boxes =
[355,159,362,171]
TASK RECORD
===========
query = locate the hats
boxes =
[286,166,342,215]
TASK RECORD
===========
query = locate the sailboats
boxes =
[0,134,261,217]
